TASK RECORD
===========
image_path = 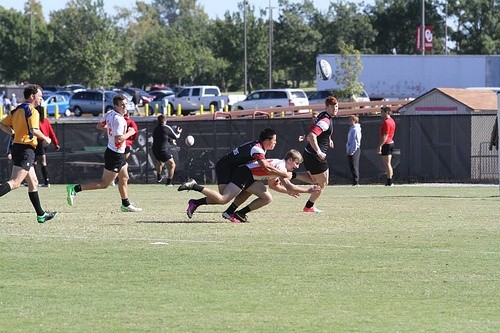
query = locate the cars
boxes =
[41,85,87,102]
[41,94,73,117]
[97,87,172,107]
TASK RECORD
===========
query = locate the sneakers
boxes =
[177,178,197,192]
[234,212,250,222]
[222,211,240,223]
[120,205,142,212]
[186,199,197,219]
[37,210,57,223]
[66,184,76,206]
[303,206,323,212]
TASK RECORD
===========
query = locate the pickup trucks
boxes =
[160,85,247,116]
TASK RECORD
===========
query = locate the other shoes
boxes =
[156,176,163,182]
[38,183,50,187]
[166,183,174,186]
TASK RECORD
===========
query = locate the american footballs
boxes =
[318,60,332,79]
[185,136,194,146]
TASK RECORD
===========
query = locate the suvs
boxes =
[68,90,135,117]
[308,86,371,114]
[229,89,310,118]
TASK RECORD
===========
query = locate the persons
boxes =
[290,96,338,213]
[152,115,182,185]
[0,84,58,223]
[346,114,362,187]
[66,94,143,212]
[3,95,11,114]
[187,128,292,223]
[10,93,17,105]
[299,112,319,141]
[131,94,151,116]
[24,105,60,187]
[110,109,138,186]
[377,105,396,186]
[177,148,321,223]
